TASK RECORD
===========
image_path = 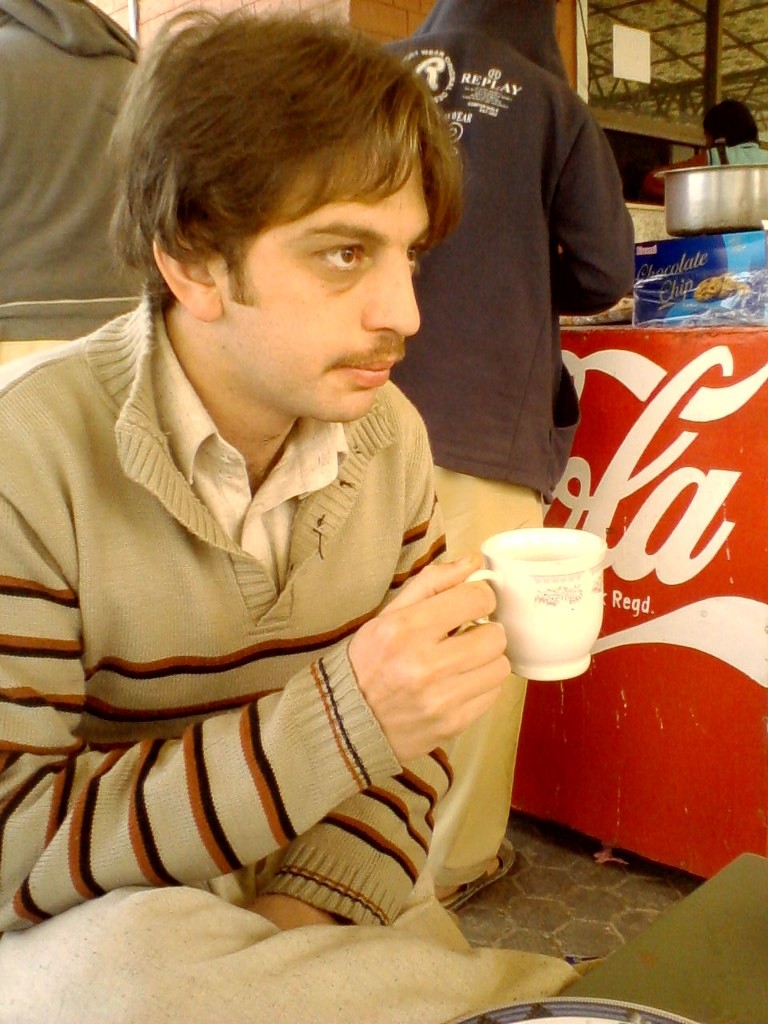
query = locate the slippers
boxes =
[438,838,516,912]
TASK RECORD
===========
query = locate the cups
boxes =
[463,527,608,682]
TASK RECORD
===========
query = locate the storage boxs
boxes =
[634,232,768,328]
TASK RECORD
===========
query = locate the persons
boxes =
[2,5,511,934]
[644,99,768,199]
[0,0,146,375]
[381,1,633,910]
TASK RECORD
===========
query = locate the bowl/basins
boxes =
[653,163,768,237]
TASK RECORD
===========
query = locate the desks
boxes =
[507,313,768,880]
